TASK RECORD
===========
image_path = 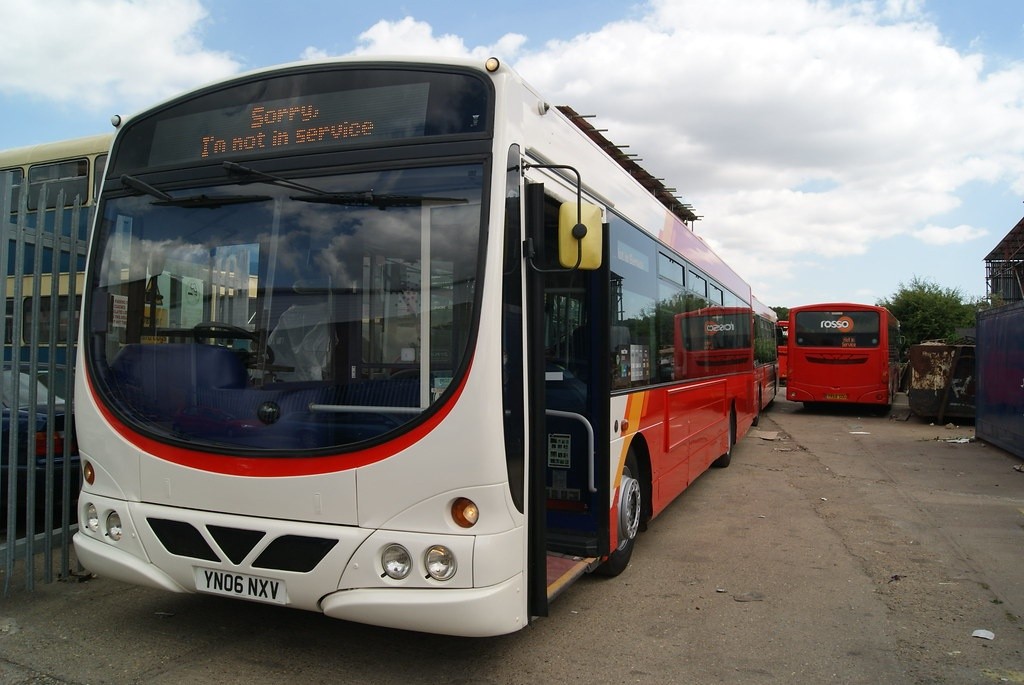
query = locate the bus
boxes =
[1,134,259,399]
[750,294,780,426]
[787,303,906,415]
[73,54,755,639]
[775,320,789,385]
[672,305,754,382]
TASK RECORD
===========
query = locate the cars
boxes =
[1,360,83,524]
